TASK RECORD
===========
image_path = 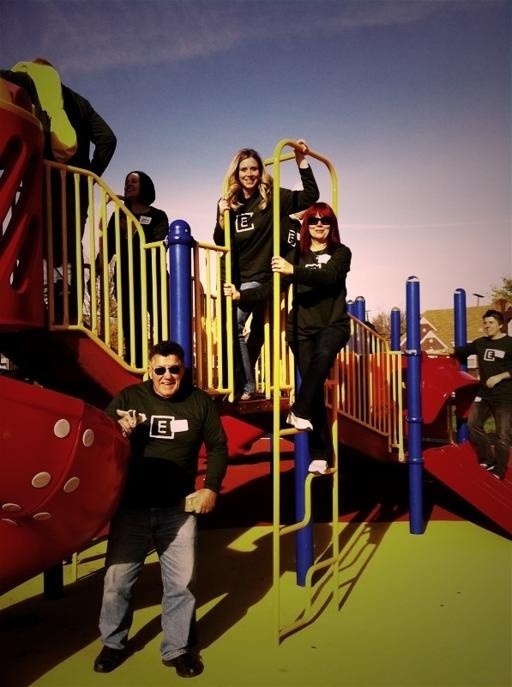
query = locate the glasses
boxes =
[155,365,179,377]
[306,216,329,224]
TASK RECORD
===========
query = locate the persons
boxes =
[86,168,171,367]
[223,200,352,476]
[93,341,230,678]
[29,55,118,326]
[426,308,512,480]
[212,139,320,404]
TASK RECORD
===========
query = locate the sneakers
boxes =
[480,461,503,479]
[308,459,329,474]
[93,645,130,672]
[162,651,204,677]
[285,413,313,431]
